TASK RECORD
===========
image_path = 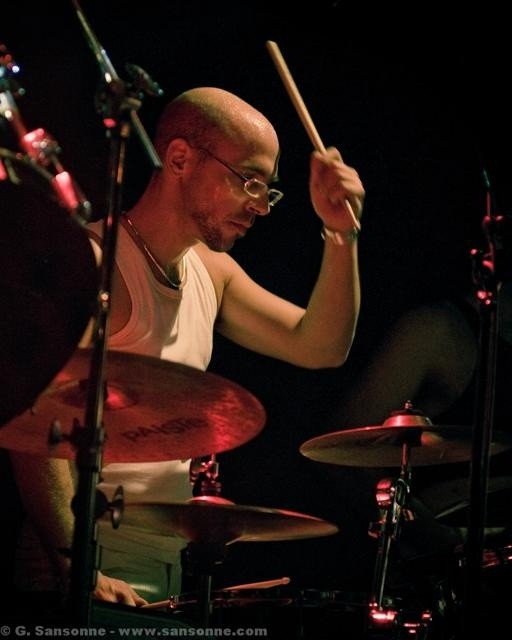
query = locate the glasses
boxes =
[192,139,284,207]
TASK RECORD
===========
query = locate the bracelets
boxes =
[320,220,363,246]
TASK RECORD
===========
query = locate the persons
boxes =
[12,83,366,620]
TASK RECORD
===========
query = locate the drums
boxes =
[1,84,102,429]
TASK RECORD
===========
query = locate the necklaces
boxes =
[121,210,187,289]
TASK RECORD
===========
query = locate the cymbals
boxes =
[2,346,265,465]
[299,414,509,468]
[97,495,341,544]
